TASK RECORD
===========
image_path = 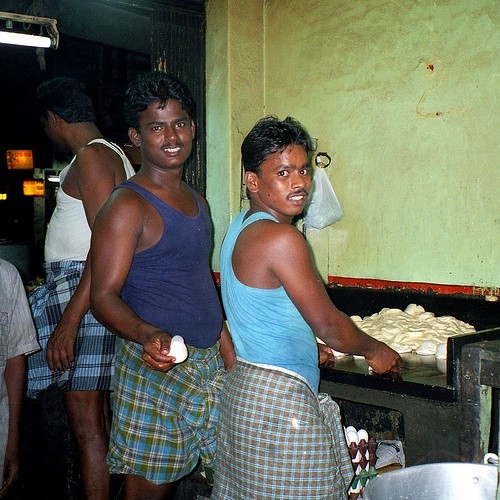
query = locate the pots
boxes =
[364,462,500,500]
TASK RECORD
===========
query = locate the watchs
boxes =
[315,153,333,168]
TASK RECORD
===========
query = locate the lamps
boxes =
[0,12,60,50]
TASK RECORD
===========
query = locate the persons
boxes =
[210,116,402,500]
[0,259,42,500]
[90,72,236,500]
[28,79,136,500]
